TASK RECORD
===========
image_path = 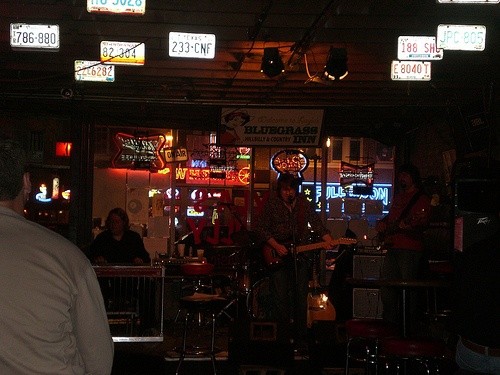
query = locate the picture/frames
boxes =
[216,106,327,148]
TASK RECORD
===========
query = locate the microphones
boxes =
[217,201,235,207]
[287,194,295,205]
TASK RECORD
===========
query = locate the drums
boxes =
[235,273,270,320]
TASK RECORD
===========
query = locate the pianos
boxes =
[93,262,167,278]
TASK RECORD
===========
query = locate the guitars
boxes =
[306,238,335,329]
[263,238,359,267]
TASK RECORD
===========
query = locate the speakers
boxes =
[309,319,349,360]
[453,216,500,254]
[353,255,386,280]
[229,307,295,367]
[353,288,386,320]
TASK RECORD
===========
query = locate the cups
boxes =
[177,244,185,256]
[197,249,203,257]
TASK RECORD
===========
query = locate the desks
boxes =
[345,278,453,338]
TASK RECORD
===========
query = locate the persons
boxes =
[88,208,154,337]
[0,139,115,375]
[257,174,333,329]
[375,163,431,325]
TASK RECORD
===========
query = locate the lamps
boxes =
[325,48,348,81]
[260,47,284,79]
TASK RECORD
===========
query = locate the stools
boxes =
[180,262,214,295]
[176,295,220,375]
[345,317,392,375]
[382,338,444,375]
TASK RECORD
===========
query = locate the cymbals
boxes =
[183,291,225,302]
